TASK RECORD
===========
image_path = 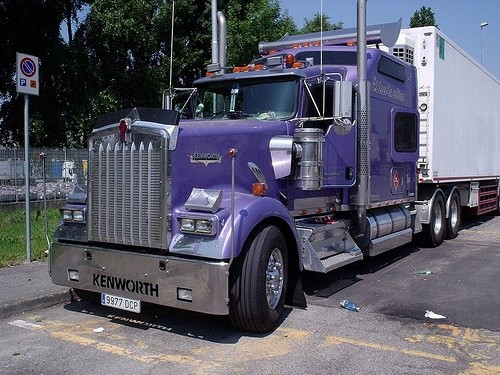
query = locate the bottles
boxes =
[339,299,360,312]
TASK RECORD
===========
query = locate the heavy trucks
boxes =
[47,16,499,339]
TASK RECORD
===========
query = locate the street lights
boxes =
[480,21,488,66]
